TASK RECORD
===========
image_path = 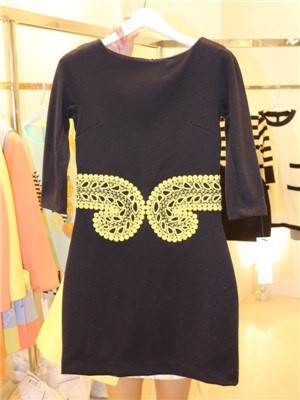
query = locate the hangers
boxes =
[101,3,200,48]
[262,84,294,116]
[20,82,48,126]
